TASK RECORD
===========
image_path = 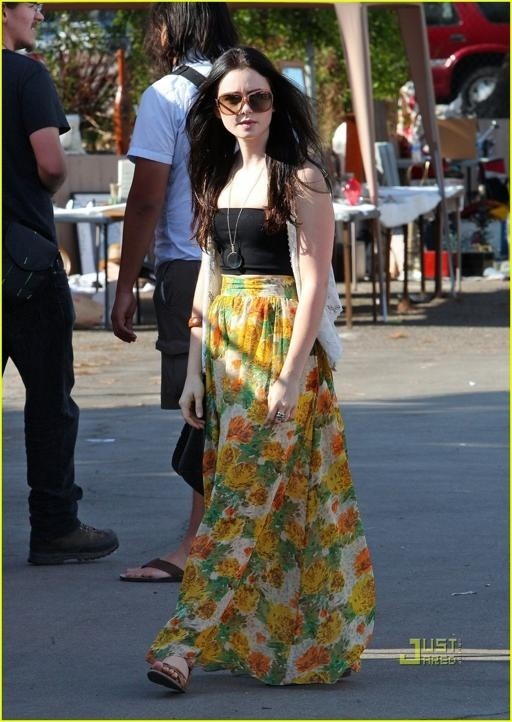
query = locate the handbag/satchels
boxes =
[2,217,60,311]
[171,371,205,497]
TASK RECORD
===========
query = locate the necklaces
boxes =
[226,152,266,271]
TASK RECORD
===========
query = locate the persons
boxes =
[3,4,118,565]
[145,49,375,693]
[111,4,239,584]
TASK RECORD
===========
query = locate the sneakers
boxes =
[27,521,118,565]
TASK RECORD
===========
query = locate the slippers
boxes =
[119,558,184,583]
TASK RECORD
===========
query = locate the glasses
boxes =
[216,88,274,116]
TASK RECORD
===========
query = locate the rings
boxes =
[274,412,287,422]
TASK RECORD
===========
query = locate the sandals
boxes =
[147,655,192,694]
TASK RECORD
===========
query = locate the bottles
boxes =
[410,135,423,162]
[108,183,121,205]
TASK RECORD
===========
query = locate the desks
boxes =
[45,177,471,337]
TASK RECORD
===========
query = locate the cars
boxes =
[398,2,510,119]
